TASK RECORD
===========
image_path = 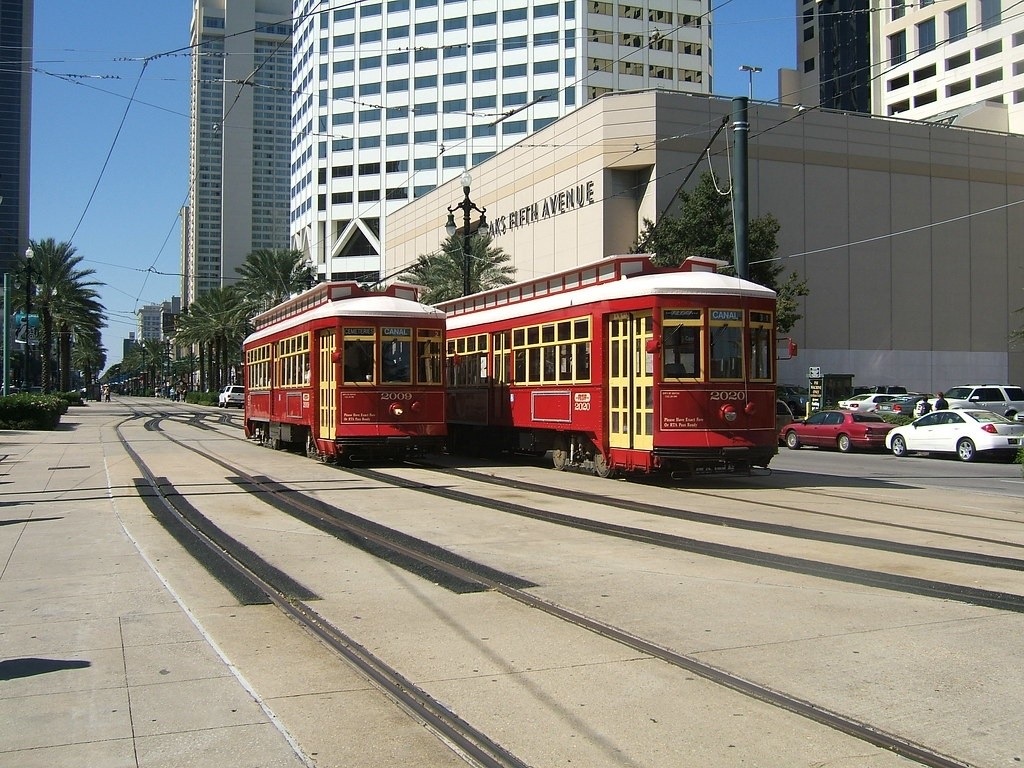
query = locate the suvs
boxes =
[913,384,1024,420]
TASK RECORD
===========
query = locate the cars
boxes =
[219,385,244,409]
[779,410,900,453]
[777,384,936,435]
[885,409,1024,462]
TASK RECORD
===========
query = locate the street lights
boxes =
[14,247,43,392]
[739,65,762,103]
[115,367,120,382]
[166,336,170,377]
[142,343,145,371]
[445,173,489,296]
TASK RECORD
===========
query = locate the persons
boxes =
[170,387,186,402]
[920,397,933,416]
[104,386,110,402]
[154,386,165,398]
[935,392,949,411]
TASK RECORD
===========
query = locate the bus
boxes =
[429,254,797,486]
[243,280,447,468]
